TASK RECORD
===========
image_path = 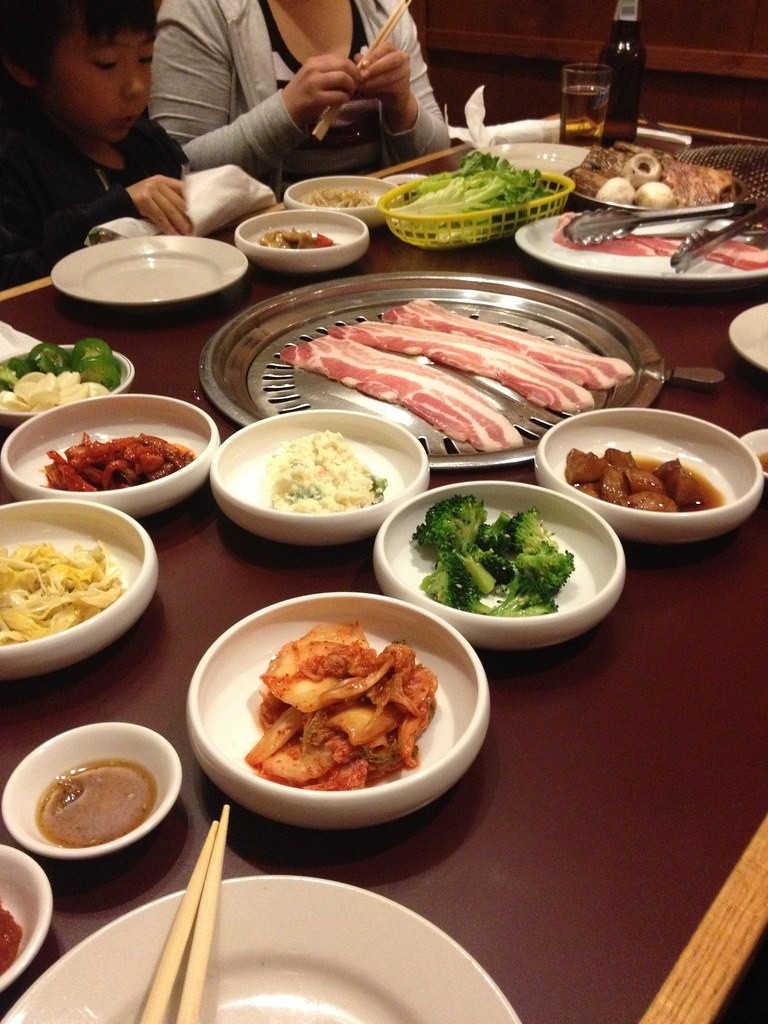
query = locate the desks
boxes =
[1,120,767,1024]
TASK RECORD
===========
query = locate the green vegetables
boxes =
[386,150,548,243]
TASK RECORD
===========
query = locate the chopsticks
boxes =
[311,0,412,142]
[134,804,230,1024]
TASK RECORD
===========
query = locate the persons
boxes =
[1,0,195,290]
[147,0,451,204]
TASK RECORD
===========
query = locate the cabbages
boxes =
[0,540,125,647]
[243,619,438,790]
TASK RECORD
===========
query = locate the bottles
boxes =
[596,0,646,147]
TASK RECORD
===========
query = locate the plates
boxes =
[0,844,54,994]
[728,302,768,372]
[739,428,768,479]
[514,211,767,293]
[467,142,589,176]
[50,235,248,305]
[0,874,522,1024]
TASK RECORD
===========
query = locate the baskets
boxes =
[378,173,575,250]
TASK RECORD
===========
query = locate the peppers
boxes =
[0,338,120,394]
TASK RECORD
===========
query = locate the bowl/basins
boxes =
[0,721,184,860]
[373,480,628,651]
[209,409,431,545]
[0,498,160,678]
[1,393,221,519]
[0,344,137,426]
[283,175,399,226]
[234,208,370,274]
[535,406,766,543]
[185,591,491,830]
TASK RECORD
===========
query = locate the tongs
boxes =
[563,198,768,275]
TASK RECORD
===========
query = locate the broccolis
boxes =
[411,493,575,617]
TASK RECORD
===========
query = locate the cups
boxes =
[559,62,612,146]
